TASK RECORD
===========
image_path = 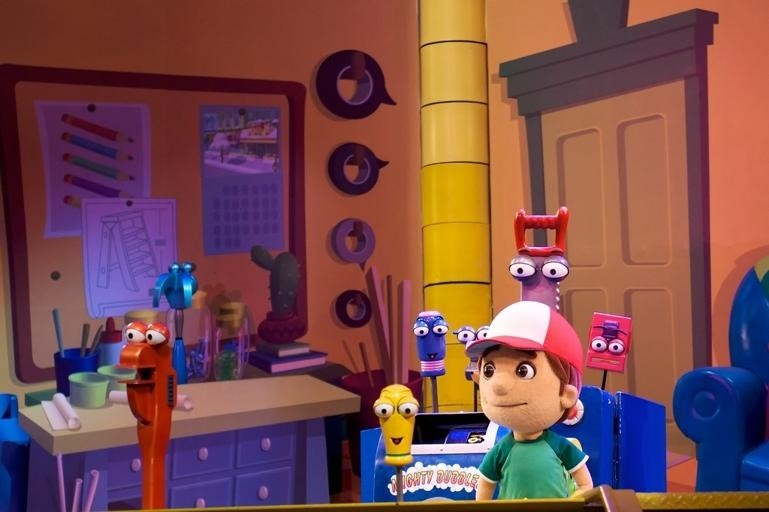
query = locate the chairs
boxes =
[671,256,768,492]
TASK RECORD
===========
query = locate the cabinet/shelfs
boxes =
[24,420,302,511]
